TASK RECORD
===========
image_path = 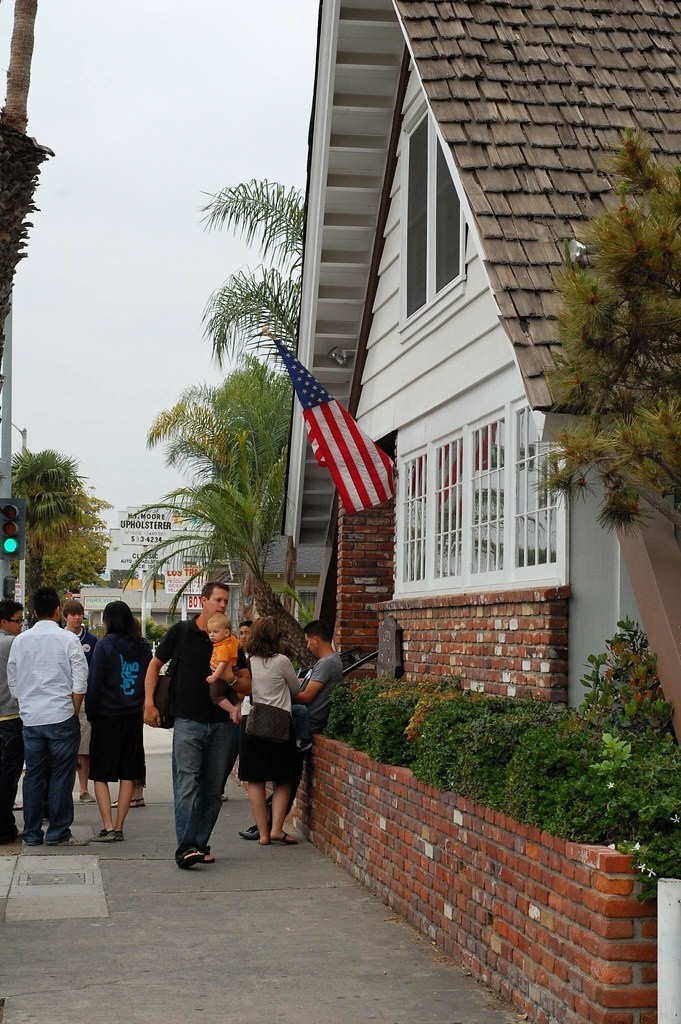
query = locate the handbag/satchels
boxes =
[245,702,292,744]
[149,619,188,729]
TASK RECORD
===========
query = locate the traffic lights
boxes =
[0,497,26,560]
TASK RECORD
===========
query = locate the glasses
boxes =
[4,618,24,624]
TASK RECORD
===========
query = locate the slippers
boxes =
[258,838,270,845]
[271,833,298,844]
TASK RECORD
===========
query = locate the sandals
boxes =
[138,798,146,807]
[111,798,138,808]
[178,849,205,869]
[203,858,215,863]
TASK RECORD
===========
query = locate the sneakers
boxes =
[115,831,125,841]
[26,838,45,846]
[47,835,90,846]
[89,829,116,841]
[80,792,95,803]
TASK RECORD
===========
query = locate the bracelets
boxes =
[228,676,238,687]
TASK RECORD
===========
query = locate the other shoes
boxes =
[239,825,260,840]
[297,739,313,753]
[221,793,228,801]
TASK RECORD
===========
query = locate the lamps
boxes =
[326,347,357,366]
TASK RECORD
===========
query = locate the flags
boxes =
[273,338,395,514]
[407,422,497,508]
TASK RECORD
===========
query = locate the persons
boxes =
[0,599,24,844]
[143,580,343,868]
[84,598,154,841]
[61,601,99,802]
[6,585,91,846]
